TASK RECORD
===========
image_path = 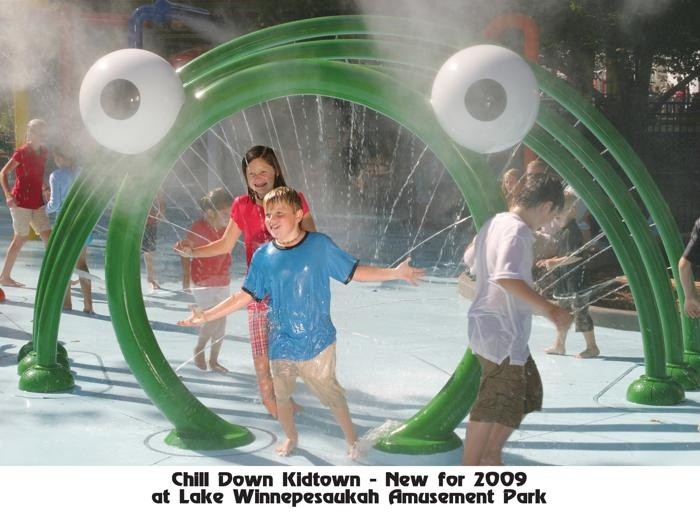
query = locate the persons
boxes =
[172,145,428,460]
[456,162,601,465]
[650,76,690,115]
[0,118,166,313]
[678,219,699,319]
